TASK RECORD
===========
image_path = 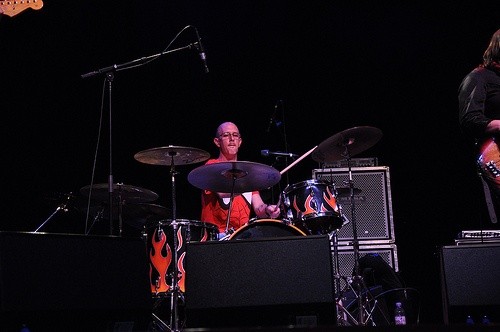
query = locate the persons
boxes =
[459,30,500,229]
[201,122,281,239]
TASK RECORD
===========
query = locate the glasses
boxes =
[216,132,241,138]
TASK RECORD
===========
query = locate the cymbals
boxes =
[86,203,169,222]
[187,161,281,194]
[134,146,211,166]
[69,183,159,206]
[311,126,383,163]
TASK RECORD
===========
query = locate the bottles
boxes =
[394,302,407,326]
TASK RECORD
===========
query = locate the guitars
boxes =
[476,131,500,189]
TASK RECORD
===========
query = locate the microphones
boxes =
[261,150,298,158]
[267,105,277,132]
[194,27,209,73]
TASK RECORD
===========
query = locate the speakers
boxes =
[313,166,406,326]
[0,232,154,332]
[438,243,499,324]
[185,234,339,328]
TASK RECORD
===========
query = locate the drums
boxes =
[342,283,420,327]
[144,218,220,300]
[280,179,344,235]
[227,218,307,241]
